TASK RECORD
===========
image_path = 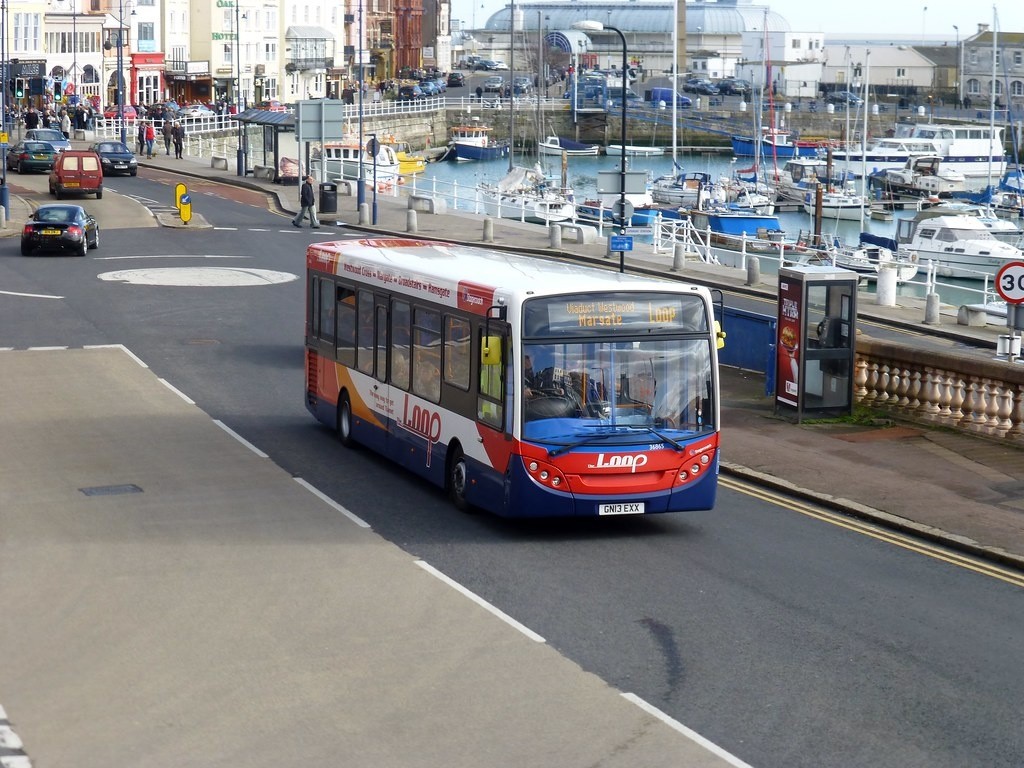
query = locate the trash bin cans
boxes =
[320,181,337,214]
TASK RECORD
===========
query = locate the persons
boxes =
[362,80,369,99]
[138,96,230,128]
[161,120,174,156]
[291,175,321,229]
[43,103,56,129]
[475,84,483,104]
[171,121,185,160]
[498,82,511,104]
[523,348,565,399]
[75,103,95,131]
[138,121,156,160]
[568,65,575,84]
[4,103,38,132]
[379,79,395,96]
[61,110,71,142]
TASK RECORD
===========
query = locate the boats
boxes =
[311,124,664,186]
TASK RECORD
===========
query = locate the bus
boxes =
[305,239,726,530]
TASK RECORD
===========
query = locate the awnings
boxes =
[327,67,348,76]
[285,25,334,41]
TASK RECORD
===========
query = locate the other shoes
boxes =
[292,220,302,227]
[313,227,320,228]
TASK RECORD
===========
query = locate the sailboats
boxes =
[478,0,1024,319]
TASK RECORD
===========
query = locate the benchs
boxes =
[408,195,447,214]
[254,165,275,181]
[549,222,599,244]
[136,142,159,155]
[332,179,358,197]
[957,304,1008,327]
[74,129,95,142]
[210,156,229,171]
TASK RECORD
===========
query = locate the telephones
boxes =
[817,316,842,348]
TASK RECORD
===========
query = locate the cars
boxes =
[104,105,137,125]
[6,140,57,174]
[89,140,138,175]
[393,57,866,113]
[148,104,167,121]
[179,105,219,122]
[253,100,287,112]
[26,128,71,152]
[21,204,98,257]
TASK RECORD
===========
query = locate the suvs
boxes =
[49,150,103,200]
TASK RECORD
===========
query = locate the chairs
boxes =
[796,229,811,247]
[114,146,119,151]
[756,228,768,239]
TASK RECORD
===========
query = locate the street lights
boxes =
[572,18,628,273]
[953,25,965,106]
[104,0,137,146]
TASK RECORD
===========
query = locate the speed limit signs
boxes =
[995,261,1024,303]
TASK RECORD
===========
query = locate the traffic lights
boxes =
[10,80,15,91]
[55,82,61,101]
[17,80,23,97]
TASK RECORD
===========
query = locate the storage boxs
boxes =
[766,231,787,242]
